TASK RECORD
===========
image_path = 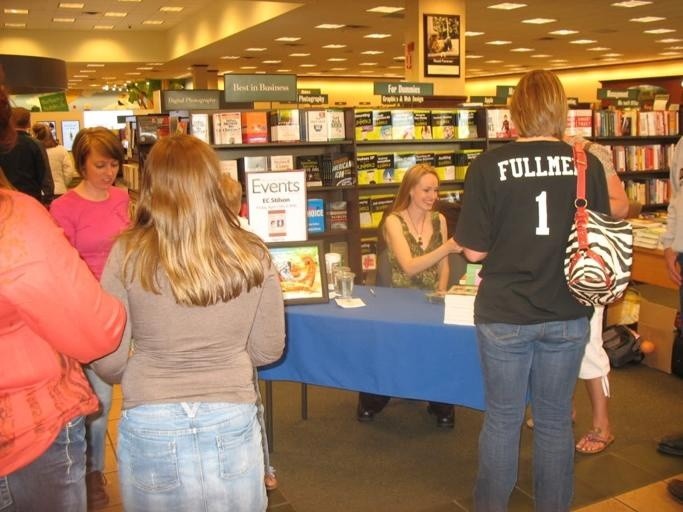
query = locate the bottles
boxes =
[323,252,341,291]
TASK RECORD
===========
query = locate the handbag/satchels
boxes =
[563,144,634,307]
[603,323,645,368]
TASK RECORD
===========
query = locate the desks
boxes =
[256,280,531,453]
[631,246,680,290]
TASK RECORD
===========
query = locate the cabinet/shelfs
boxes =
[599,76,683,109]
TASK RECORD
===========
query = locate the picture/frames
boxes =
[34,120,56,141]
[423,13,460,77]
[60,119,81,151]
[265,240,329,305]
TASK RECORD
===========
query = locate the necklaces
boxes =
[408,205,426,246]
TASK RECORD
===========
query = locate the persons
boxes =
[215,173,279,491]
[46,126,142,283]
[655,135,683,505]
[354,163,460,428]
[30,122,74,202]
[523,133,631,455]
[0,107,55,204]
[87,136,285,512]
[0,65,127,512]
[458,66,631,512]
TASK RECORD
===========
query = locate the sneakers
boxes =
[264,464,276,491]
[87,471,110,508]
[666,479,682,504]
[656,432,682,456]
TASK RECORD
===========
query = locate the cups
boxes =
[334,272,354,301]
[331,264,350,295]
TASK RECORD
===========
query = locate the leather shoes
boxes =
[437,407,455,428]
[357,402,374,423]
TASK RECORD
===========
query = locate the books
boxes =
[242,112,268,145]
[332,242,350,269]
[415,111,432,139]
[326,201,349,231]
[625,176,683,204]
[438,153,456,180]
[128,198,138,223]
[131,112,173,145]
[604,138,682,171]
[218,159,238,184]
[269,109,300,143]
[327,111,345,141]
[307,199,325,234]
[173,113,210,145]
[457,149,487,179]
[355,108,373,140]
[457,110,480,139]
[295,153,321,187]
[271,156,294,173]
[212,113,242,144]
[374,110,394,140]
[244,157,268,172]
[391,111,414,141]
[375,152,393,186]
[122,161,146,195]
[356,153,378,185]
[432,112,455,139]
[626,211,675,251]
[321,153,358,186]
[302,111,327,141]
[394,152,416,184]
[417,153,436,180]
[444,283,478,327]
[568,108,679,138]
[437,190,465,202]
[357,194,396,228]
[487,110,513,138]
[361,239,378,269]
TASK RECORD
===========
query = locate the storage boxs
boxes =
[637,297,677,374]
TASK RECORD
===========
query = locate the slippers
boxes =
[575,432,615,454]
